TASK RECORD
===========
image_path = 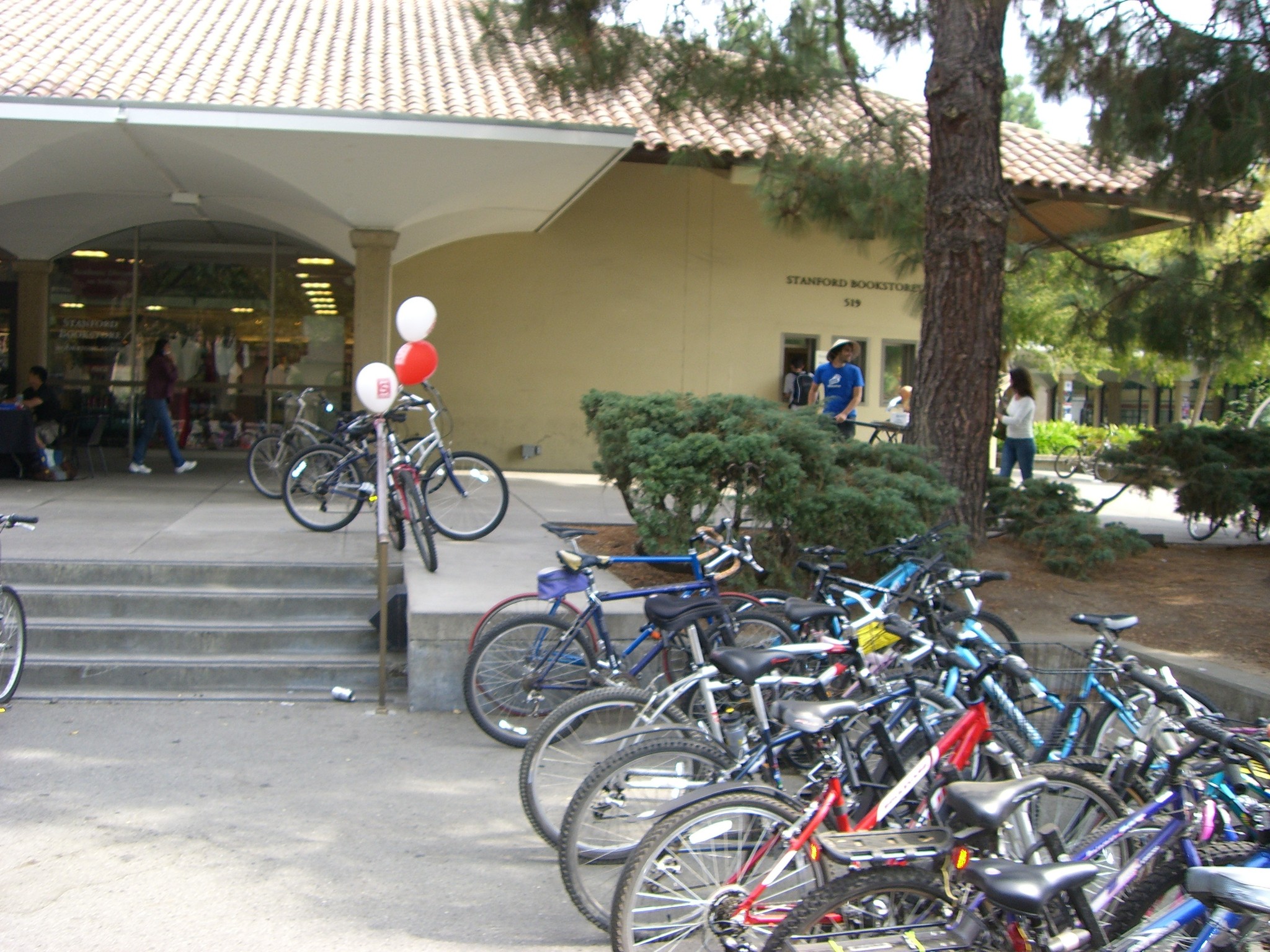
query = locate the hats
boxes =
[827,339,861,361]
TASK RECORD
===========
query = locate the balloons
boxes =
[397,296,438,341]
[355,363,397,412]
[396,341,435,384]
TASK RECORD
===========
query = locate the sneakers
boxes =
[128,462,153,474]
[175,461,198,474]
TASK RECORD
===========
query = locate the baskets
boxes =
[987,640,1115,750]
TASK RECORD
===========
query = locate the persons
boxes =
[128,337,196,472]
[996,366,1035,487]
[258,352,295,399]
[11,367,65,479]
[109,321,242,413]
[783,357,819,412]
[808,338,864,442]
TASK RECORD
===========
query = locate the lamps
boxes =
[728,161,761,187]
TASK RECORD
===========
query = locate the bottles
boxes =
[721,706,754,762]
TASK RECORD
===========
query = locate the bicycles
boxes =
[458,511,1270,952]
[1185,503,1270,543]
[0,512,42,710]
[245,377,511,573]
[1053,432,1122,483]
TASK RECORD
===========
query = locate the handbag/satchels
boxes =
[992,408,1008,442]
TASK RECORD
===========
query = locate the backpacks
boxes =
[788,370,817,409]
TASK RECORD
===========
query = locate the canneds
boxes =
[331,686,356,702]
